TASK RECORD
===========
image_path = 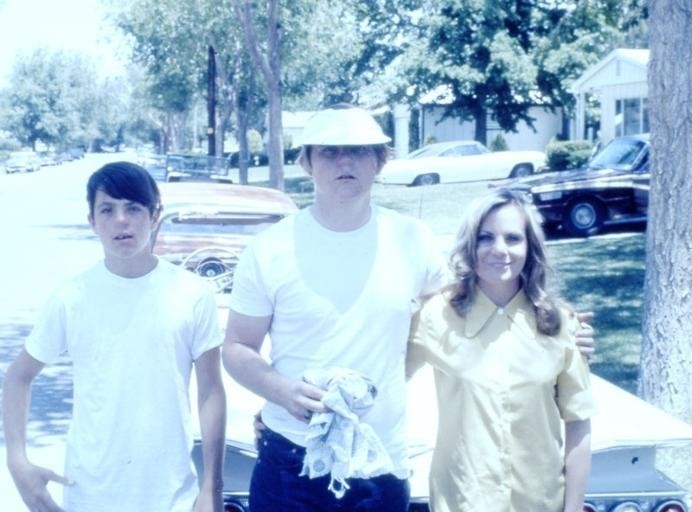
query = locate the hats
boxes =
[302,102,392,147]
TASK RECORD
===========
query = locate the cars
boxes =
[144,177,302,280]
[163,152,231,185]
[374,138,550,190]
[487,133,651,235]
[5,145,85,174]
[129,207,689,509]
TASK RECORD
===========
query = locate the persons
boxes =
[3,161,226,511]
[252,185,594,512]
[222,103,596,510]
